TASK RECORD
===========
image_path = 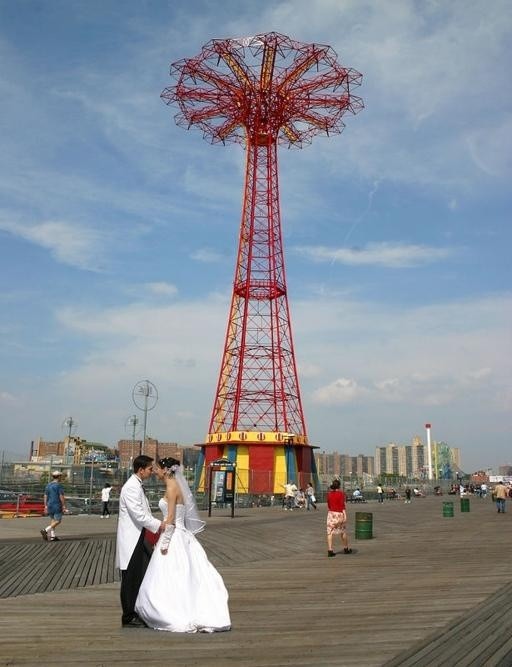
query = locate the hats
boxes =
[52,469,62,476]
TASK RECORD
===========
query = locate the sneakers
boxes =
[100,515,110,519]
[328,548,351,557]
[51,536,60,541]
[41,528,48,541]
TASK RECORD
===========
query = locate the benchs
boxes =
[280,490,456,508]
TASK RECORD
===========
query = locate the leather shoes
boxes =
[122,617,147,629]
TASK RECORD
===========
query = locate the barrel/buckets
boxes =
[442,500,454,518]
[460,498,471,511]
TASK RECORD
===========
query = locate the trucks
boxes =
[0,494,45,517]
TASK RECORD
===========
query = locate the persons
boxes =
[113,453,177,628]
[39,470,67,541]
[305,481,317,509]
[326,479,353,557]
[296,487,309,509]
[100,482,114,518]
[133,455,231,633]
[492,480,508,512]
[391,488,400,499]
[434,481,482,495]
[375,483,385,504]
[404,485,412,503]
[279,478,298,510]
[413,487,424,496]
[352,488,361,503]
[479,481,488,496]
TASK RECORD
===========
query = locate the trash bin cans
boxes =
[461,499,469,512]
[443,502,453,517]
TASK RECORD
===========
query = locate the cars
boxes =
[61,498,119,515]
[211,494,271,508]
